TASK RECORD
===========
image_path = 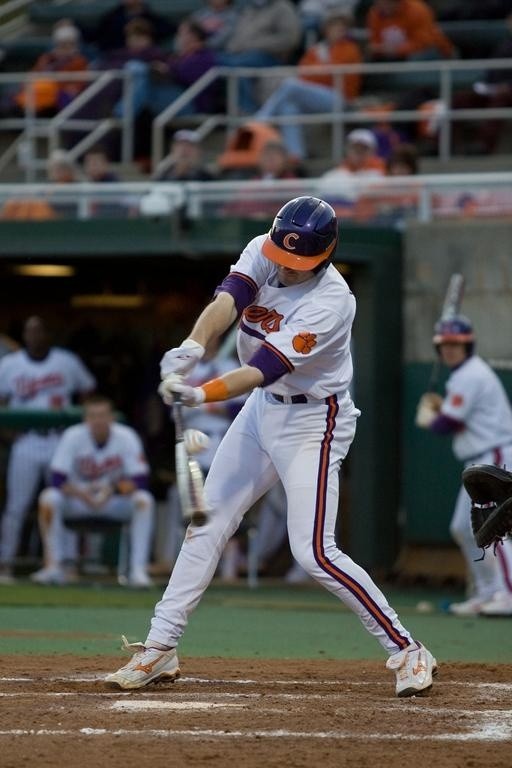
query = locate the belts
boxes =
[271,392,307,403]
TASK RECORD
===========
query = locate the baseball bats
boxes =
[416,273,462,426]
[172,391,208,527]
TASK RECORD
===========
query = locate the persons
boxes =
[0,1,510,617]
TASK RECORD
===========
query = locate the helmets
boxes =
[432,313,475,343]
[262,195,339,270]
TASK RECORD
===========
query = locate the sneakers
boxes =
[105,644,181,690]
[450,592,512,616]
[386,639,438,696]
[34,570,150,589]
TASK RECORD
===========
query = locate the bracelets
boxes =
[101,196,441,701]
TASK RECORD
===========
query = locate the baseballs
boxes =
[184,427,207,453]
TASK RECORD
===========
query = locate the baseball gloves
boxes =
[463,462,510,561]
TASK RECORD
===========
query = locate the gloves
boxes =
[160,339,205,407]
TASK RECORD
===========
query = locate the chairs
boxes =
[0,0,512,162]
[62,514,122,578]
